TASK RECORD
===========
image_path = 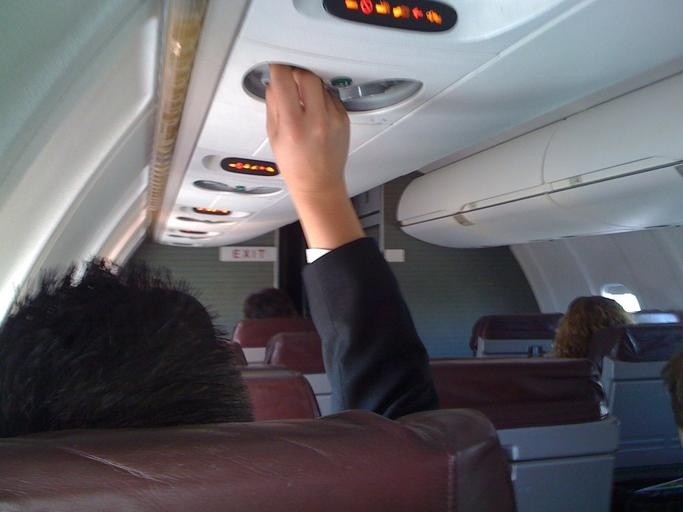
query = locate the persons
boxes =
[243,286,299,319]
[660,352,683,443]
[552,296,635,365]
[0,65,438,430]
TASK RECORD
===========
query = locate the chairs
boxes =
[0,312,681,511]
